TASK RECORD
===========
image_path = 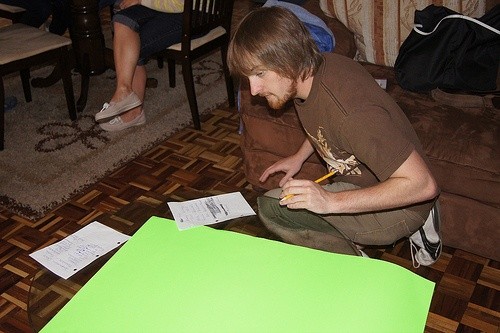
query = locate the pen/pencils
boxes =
[281,169,339,201]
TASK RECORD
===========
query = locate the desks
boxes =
[27,195,361,333]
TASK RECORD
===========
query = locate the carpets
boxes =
[0,32,228,221]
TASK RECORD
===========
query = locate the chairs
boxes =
[0,0,235,151]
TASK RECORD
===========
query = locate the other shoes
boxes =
[99,109,147,132]
[95,90,142,123]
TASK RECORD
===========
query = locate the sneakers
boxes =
[408,200,443,269]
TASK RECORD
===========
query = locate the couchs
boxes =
[241,0,500,262]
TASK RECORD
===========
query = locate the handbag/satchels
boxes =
[394,4,500,96]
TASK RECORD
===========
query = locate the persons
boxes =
[94,0,225,132]
[227,6,442,268]
